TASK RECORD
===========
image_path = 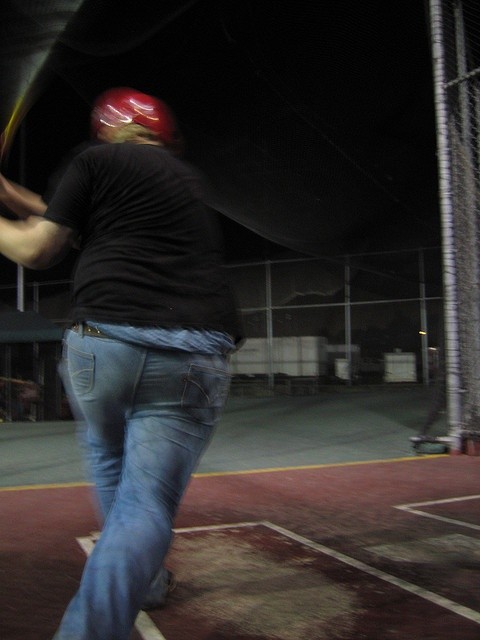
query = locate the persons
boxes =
[0,86,245,640]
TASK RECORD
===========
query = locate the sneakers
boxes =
[144,569,177,609]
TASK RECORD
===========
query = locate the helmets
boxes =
[90,90,174,141]
